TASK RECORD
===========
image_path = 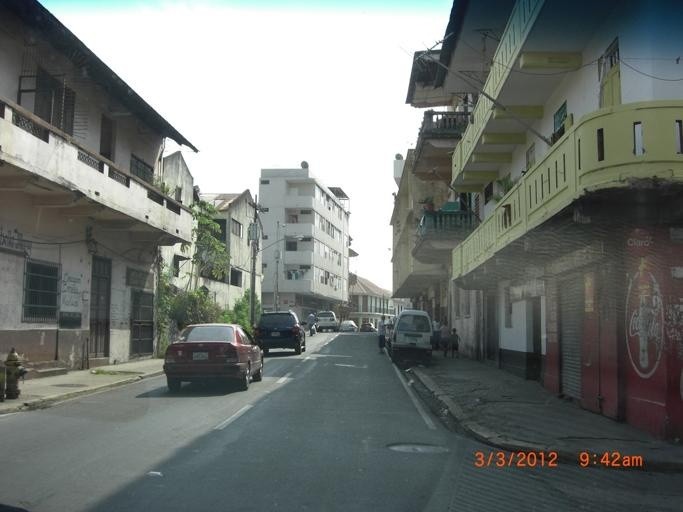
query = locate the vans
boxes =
[388,309,434,360]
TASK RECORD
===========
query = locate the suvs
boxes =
[255,310,306,354]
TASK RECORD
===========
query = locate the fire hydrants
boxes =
[4,347,28,401]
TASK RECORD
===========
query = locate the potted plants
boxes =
[418,196,435,213]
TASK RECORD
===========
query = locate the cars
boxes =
[315,309,378,334]
[162,321,264,389]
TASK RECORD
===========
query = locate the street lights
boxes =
[250,234,313,326]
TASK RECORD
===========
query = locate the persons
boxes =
[432,314,461,359]
[376,315,384,354]
[307,312,314,337]
[387,316,391,324]
